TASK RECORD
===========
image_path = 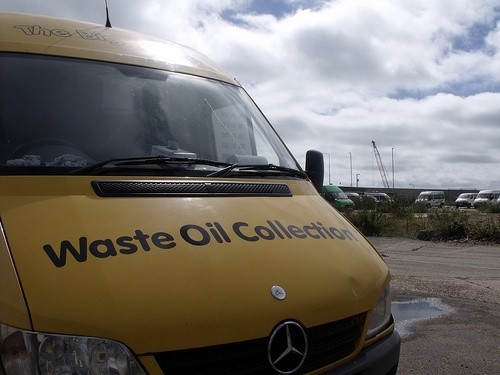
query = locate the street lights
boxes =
[356,174,360,187]
[409,184,414,189]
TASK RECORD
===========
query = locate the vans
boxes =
[474,189,500,208]
[415,191,446,209]
[455,193,478,208]
[1,0,401,375]
[346,192,395,203]
[320,184,355,211]
